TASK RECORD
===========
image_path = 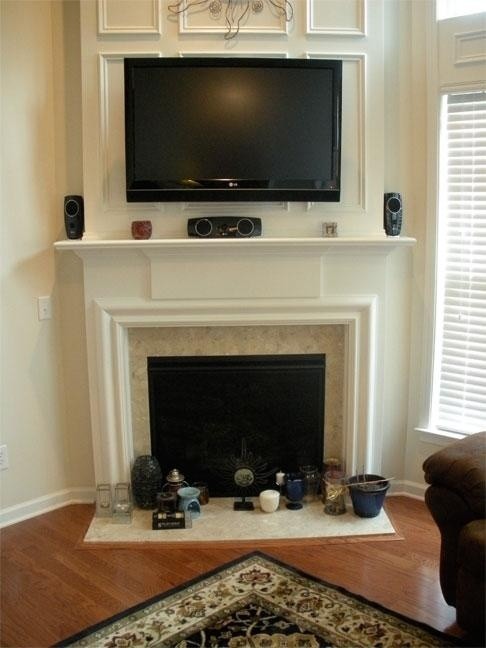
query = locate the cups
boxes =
[260,458,348,515]
[131,219,152,239]
[156,482,209,520]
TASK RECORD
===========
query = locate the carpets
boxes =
[42,547,468,647]
[73,482,410,551]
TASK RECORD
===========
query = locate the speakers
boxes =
[63,195,83,238]
[187,216,262,238]
[384,193,403,235]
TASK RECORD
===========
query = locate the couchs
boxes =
[421,427,486,636]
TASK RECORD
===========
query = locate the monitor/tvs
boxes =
[122,56,342,202]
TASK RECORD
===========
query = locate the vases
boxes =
[321,470,349,517]
[297,465,321,503]
[345,470,395,522]
[129,453,164,515]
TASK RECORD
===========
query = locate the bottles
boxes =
[131,454,167,510]
[93,481,134,524]
[164,470,190,496]
[151,511,193,529]
